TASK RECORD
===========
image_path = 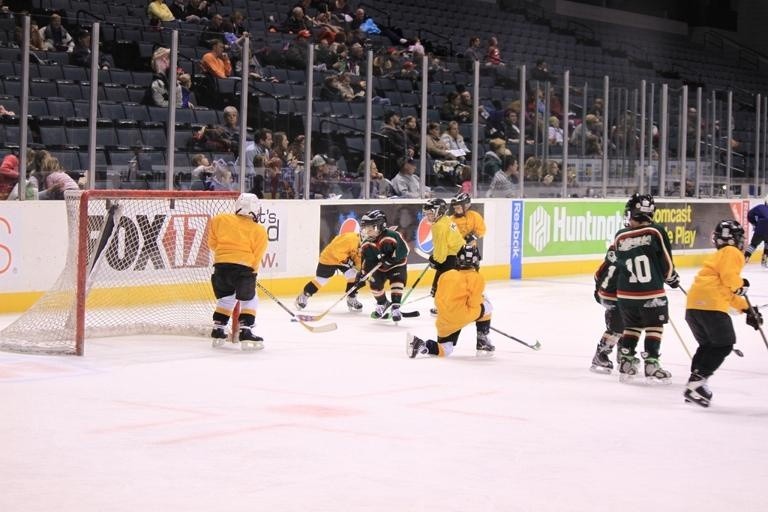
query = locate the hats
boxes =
[313,154,336,166]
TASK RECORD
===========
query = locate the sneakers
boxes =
[211,328,228,340]
[410,336,425,358]
[591,348,712,402]
[477,336,495,353]
[239,329,263,343]
[297,293,438,323]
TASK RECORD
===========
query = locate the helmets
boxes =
[623,192,655,226]
[360,193,481,270]
[713,220,747,250]
[234,193,261,223]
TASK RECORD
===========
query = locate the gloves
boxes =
[666,271,680,288]
[734,279,749,296]
[746,307,762,330]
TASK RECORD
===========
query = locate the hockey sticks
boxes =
[677,283,745,357]
[255,280,338,333]
[371,263,429,320]
[296,261,382,321]
[489,326,540,351]
[384,297,420,317]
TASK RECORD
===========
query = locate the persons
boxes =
[408,193,496,359]
[0,0,113,71]
[125,139,154,183]
[462,35,706,201]
[354,209,408,322]
[683,218,764,408]
[0,104,82,200]
[592,245,640,369]
[207,192,269,342]
[614,192,681,380]
[295,226,369,310]
[744,195,768,265]
[143,0,513,201]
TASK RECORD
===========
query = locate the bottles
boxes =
[78,173,87,189]
